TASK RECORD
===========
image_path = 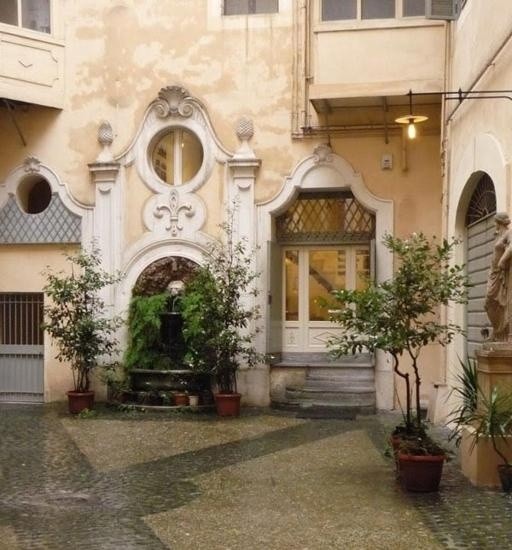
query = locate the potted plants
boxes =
[38,241,128,414]
[317,230,472,491]
[444,351,512,492]
[181,231,267,417]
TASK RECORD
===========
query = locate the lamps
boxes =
[395,90,429,140]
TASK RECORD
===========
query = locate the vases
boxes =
[188,396,199,405]
[174,394,187,405]
